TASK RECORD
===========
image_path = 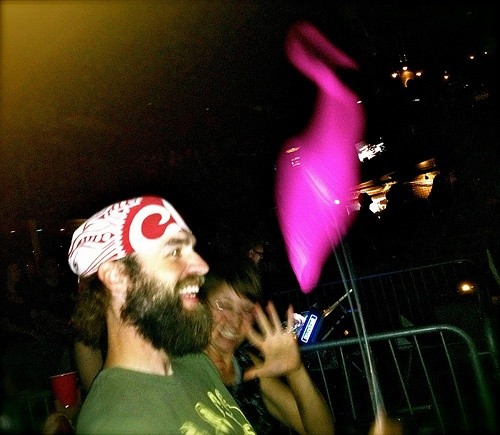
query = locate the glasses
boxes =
[204,298,255,313]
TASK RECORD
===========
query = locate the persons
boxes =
[190,257,339,435]
[351,156,462,248]
[0,233,102,435]
[65,193,257,434]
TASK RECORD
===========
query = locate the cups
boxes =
[50,371,77,409]
